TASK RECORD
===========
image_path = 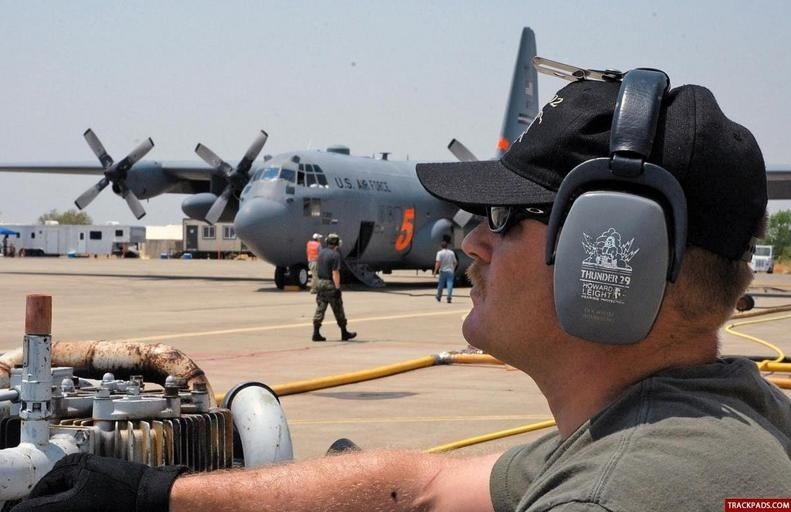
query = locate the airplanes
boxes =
[2,28,791,310]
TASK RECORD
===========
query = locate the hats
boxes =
[415,78,767,263]
[327,233,340,244]
[312,233,323,240]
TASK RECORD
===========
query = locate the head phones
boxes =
[543,67,694,348]
[324,233,342,247]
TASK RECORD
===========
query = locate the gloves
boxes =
[335,289,341,299]
[9,452,193,512]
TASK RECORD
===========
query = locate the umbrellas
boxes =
[0,227,16,234]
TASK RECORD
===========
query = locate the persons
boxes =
[10,78,789,512]
[432,239,459,303]
[305,232,321,294]
[311,233,357,340]
[1,235,8,256]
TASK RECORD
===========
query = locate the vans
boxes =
[752,244,776,273]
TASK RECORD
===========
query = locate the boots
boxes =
[312,326,326,341]
[341,327,357,341]
